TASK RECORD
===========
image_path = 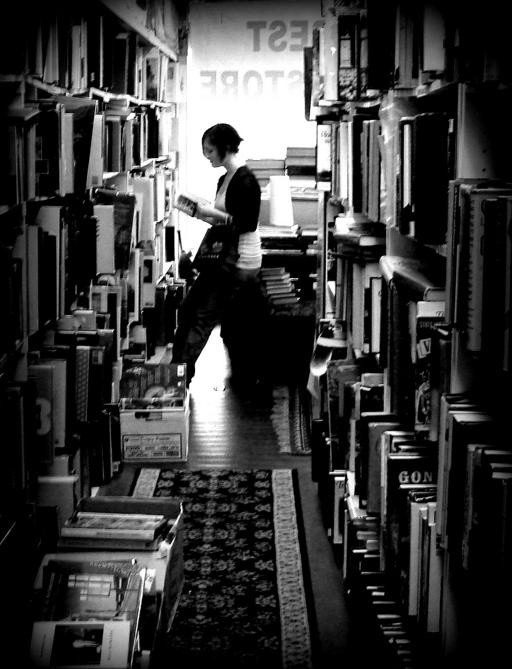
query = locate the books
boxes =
[1,5,196,664]
[246,3,512,669]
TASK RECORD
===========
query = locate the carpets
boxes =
[269,378,312,453]
[131,466,320,663]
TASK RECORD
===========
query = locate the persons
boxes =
[174,123,263,387]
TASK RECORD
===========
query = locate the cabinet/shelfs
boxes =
[304,85,510,665]
[0,4,182,592]
